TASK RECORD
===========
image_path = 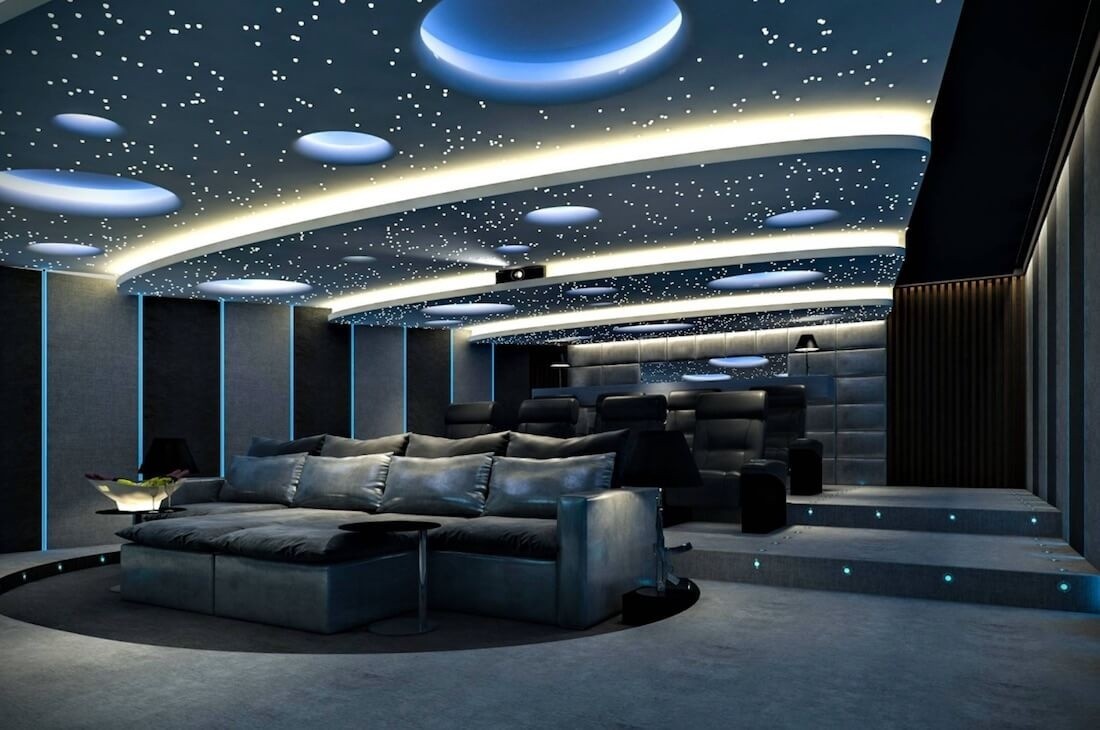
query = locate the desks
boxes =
[96,508,186,592]
[339,521,444,636]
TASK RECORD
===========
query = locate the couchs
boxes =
[116,430,663,628]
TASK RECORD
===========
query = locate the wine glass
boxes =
[149,479,159,513]
[165,479,175,512]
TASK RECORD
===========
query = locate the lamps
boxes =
[551,351,571,388]
[795,334,820,376]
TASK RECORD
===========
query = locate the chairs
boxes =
[665,389,723,451]
[692,391,770,512]
[518,399,578,438]
[602,394,713,524]
[750,385,808,470]
[443,401,499,438]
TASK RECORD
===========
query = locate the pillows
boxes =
[219,452,309,503]
[376,452,494,518]
[292,452,392,512]
[482,450,616,517]
